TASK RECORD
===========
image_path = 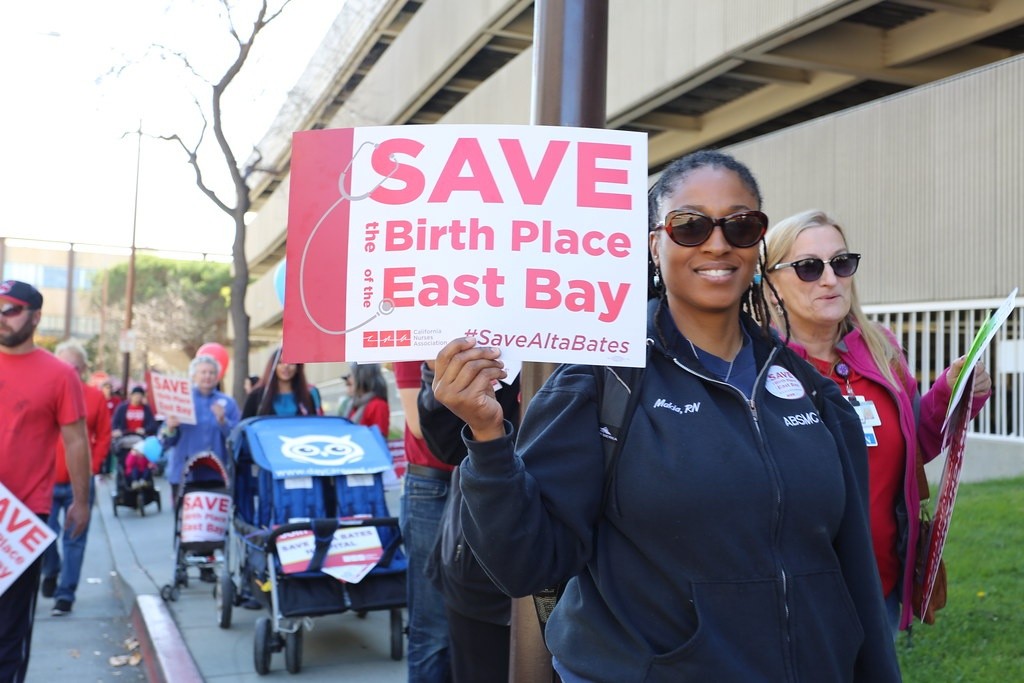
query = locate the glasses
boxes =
[654,208,769,248]
[0,303,23,316]
[774,253,861,282]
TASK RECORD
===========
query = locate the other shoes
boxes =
[42,572,57,597]
[200,568,217,582]
[52,600,71,615]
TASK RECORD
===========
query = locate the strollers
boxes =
[158,450,233,604]
[107,428,162,516]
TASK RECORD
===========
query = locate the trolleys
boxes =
[218,408,413,674]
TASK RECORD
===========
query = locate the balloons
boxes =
[194,343,229,382]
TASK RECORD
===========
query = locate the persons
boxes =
[331,362,390,444]
[41,337,123,617]
[110,386,158,494]
[430,149,905,683]
[239,346,325,611]
[392,360,520,683]
[0,281,93,683]
[158,354,241,586]
[125,444,157,487]
[759,209,992,646]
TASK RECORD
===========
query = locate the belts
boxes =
[408,463,451,482]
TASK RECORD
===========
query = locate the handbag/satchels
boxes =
[912,519,947,624]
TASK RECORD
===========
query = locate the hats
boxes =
[0,280,42,308]
